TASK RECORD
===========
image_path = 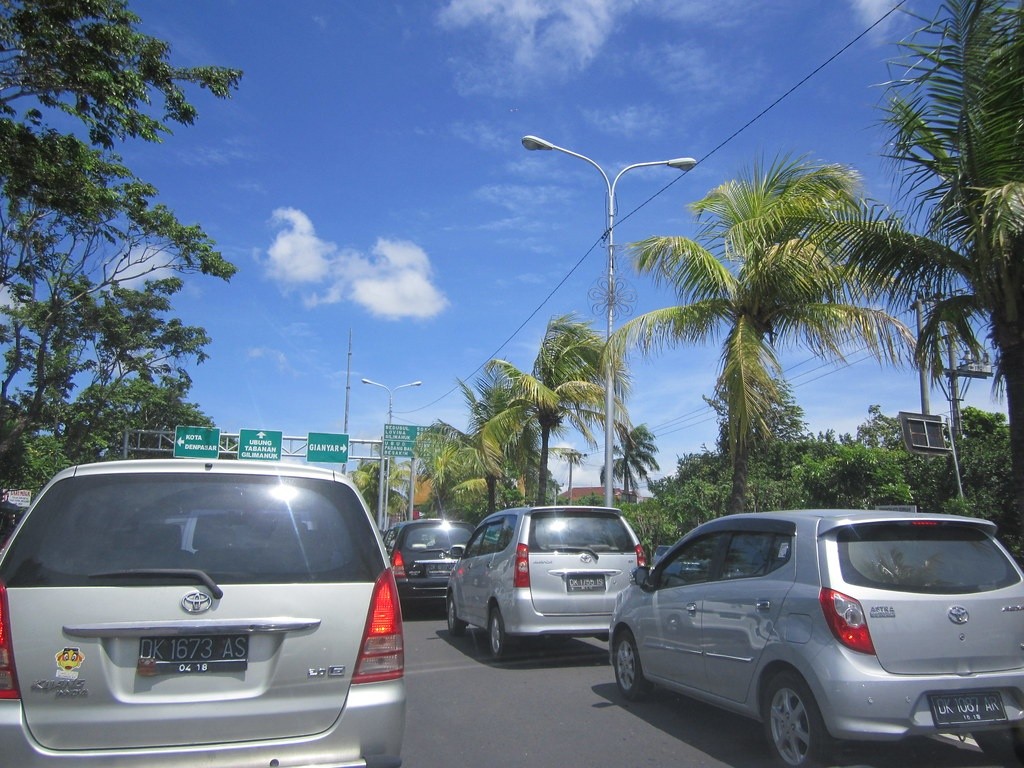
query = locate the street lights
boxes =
[361,377,422,529]
[521,133,712,508]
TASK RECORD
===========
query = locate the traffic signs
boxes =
[307,432,350,463]
[173,426,220,460]
[237,428,282,461]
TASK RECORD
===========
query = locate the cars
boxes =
[445,506,648,662]
[383,519,476,615]
[0,458,407,768]
[609,508,1024,768]
